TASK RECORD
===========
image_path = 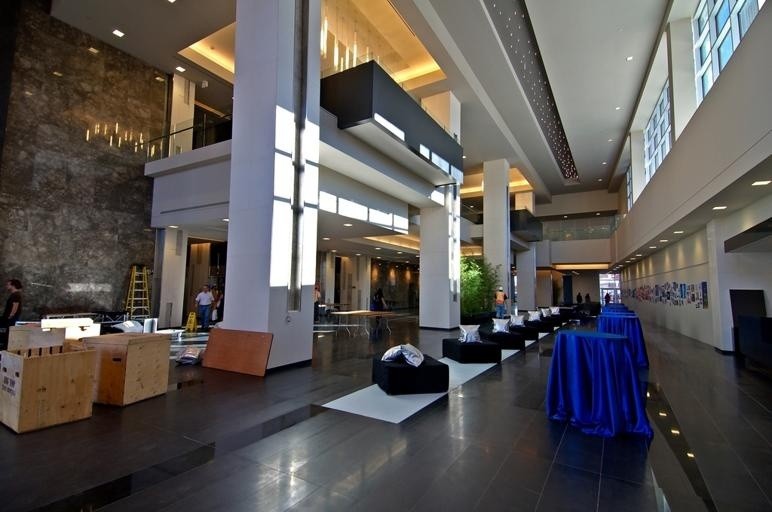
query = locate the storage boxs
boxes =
[0,345,93,435]
[79,330,170,411]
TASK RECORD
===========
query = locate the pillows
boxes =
[381,343,402,362]
[528,310,541,322]
[551,306,560,315]
[400,343,425,367]
[491,317,511,334]
[542,308,551,317]
[459,325,482,343]
[510,314,526,328]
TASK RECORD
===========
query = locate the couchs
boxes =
[511,327,539,340]
[443,337,502,364]
[524,320,554,333]
[541,315,562,328]
[372,353,449,395]
[479,329,525,352]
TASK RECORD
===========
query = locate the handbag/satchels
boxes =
[211,309,217,320]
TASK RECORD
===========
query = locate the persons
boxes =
[494,287,508,319]
[0,278,23,350]
[194,285,215,332]
[605,293,610,305]
[585,294,590,303]
[314,284,322,322]
[577,293,582,304]
[373,288,387,320]
[209,284,224,324]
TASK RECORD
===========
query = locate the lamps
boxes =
[320,0,380,73]
[84,120,156,157]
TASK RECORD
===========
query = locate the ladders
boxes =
[125,263,151,320]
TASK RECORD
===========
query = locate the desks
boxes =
[596,314,649,369]
[318,303,351,324]
[546,329,649,441]
[602,303,635,315]
[331,310,394,340]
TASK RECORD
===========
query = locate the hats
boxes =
[499,287,503,290]
